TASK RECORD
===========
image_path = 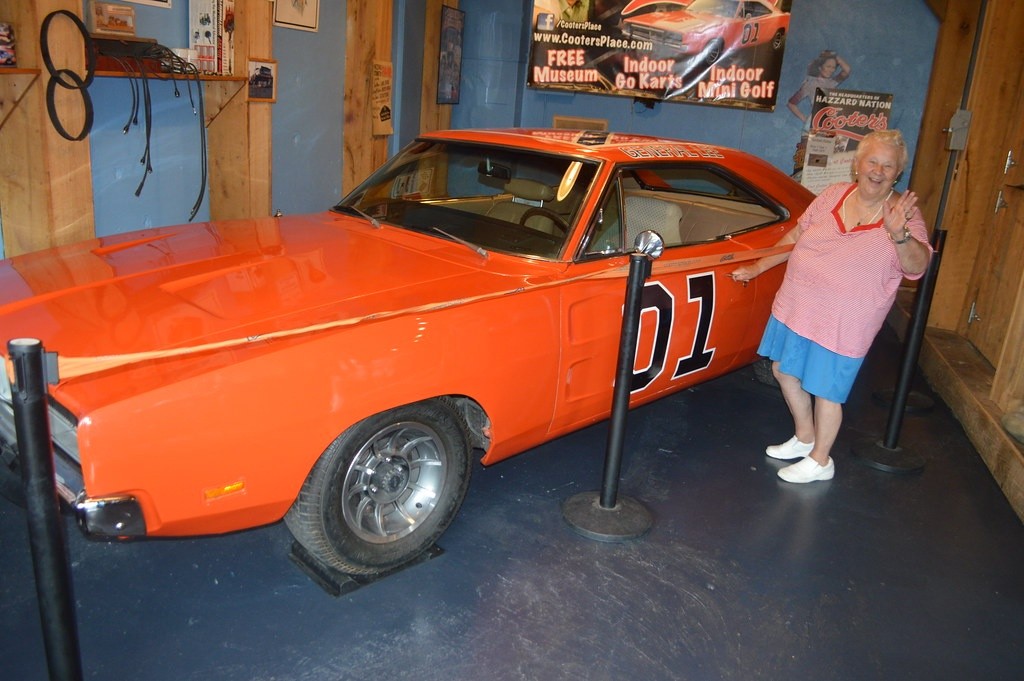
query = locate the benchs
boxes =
[650,193,777,242]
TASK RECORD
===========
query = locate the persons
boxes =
[787,51,850,171]
[732,130,933,483]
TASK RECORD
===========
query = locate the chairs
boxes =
[472,176,554,257]
[587,194,683,251]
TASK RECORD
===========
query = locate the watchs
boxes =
[890,226,911,244]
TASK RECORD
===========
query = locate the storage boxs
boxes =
[188,0,234,77]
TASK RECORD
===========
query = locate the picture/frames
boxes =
[245,57,277,103]
[90,0,136,36]
[273,0,319,33]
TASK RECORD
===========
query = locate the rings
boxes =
[904,209,909,213]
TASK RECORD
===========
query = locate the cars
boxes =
[0,128,820,576]
[622,0,790,68]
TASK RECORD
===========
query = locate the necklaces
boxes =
[842,188,893,229]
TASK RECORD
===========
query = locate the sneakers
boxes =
[765,435,815,460]
[777,456,835,483]
